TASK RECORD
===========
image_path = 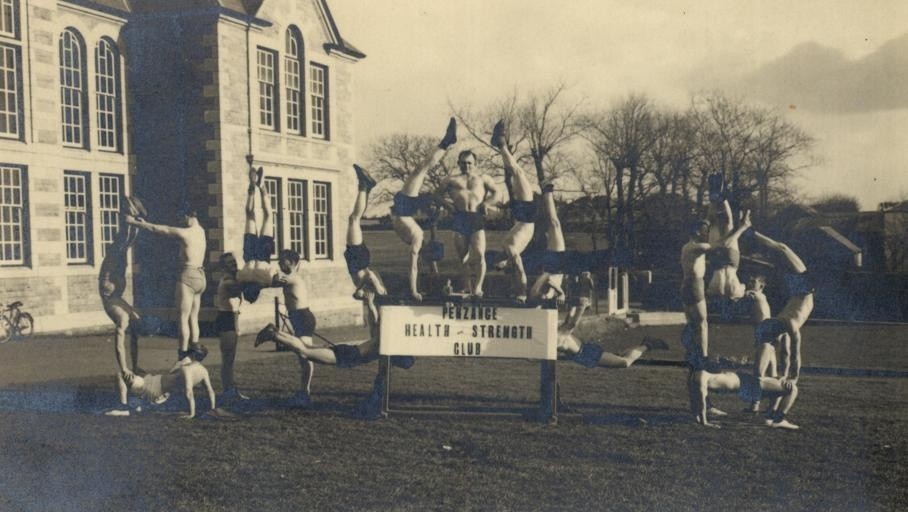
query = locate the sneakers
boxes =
[708,172,724,203]
[120,195,148,218]
[255,324,277,347]
[447,117,457,143]
[490,118,505,145]
[642,335,669,350]
[766,418,801,430]
[222,387,311,408]
[354,164,377,191]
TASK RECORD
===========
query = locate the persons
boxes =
[678,173,814,429]
[99,194,218,421]
[391,117,535,304]
[527,183,673,369]
[214,153,412,404]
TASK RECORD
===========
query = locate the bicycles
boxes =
[0,300,34,344]
